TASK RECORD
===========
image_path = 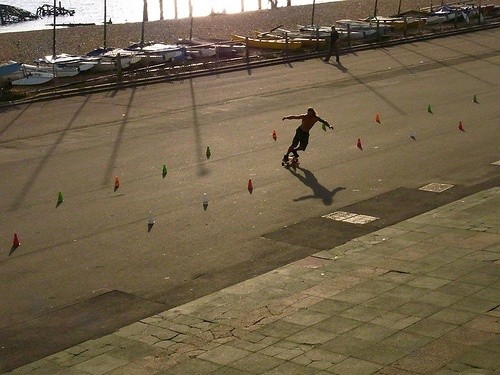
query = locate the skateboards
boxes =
[282,155,296,165]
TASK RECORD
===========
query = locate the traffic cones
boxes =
[13,234,20,246]
[57,191,63,201]
[203,193,209,204]
[147,211,156,225]
[162,164,167,178]
[357,139,361,147]
[375,113,381,122]
[114,176,120,187]
[322,123,326,130]
[248,179,254,190]
[459,120,463,129]
[206,145,211,159]
[272,129,277,140]
[409,128,416,137]
[428,104,432,112]
[472,94,477,102]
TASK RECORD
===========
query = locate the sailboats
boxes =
[358,0,427,28]
[87,0,145,71]
[123,0,185,66]
[179,17,216,60]
[296,0,375,40]
[8,0,102,77]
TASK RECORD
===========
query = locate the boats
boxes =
[277,28,348,44]
[0,63,57,85]
[178,39,246,57]
[427,0,500,25]
[335,19,395,29]
[0,60,32,86]
[254,30,326,47]
[229,33,302,50]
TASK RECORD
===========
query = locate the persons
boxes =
[324,26,339,62]
[282,107,334,161]
